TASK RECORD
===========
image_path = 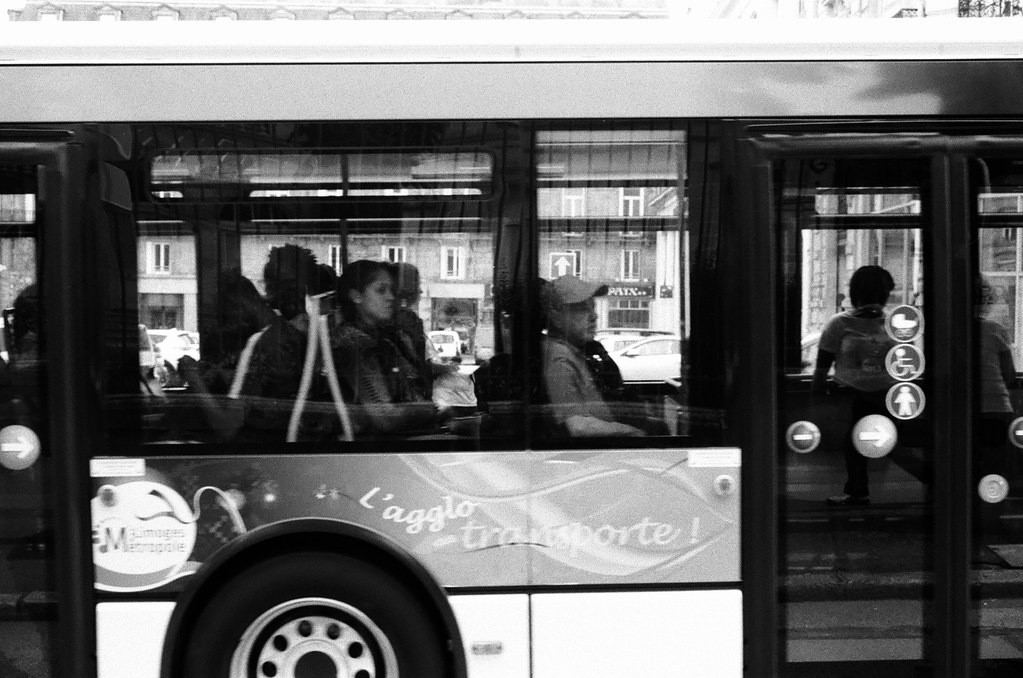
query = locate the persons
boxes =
[809,265,932,506]
[179,246,453,443]
[970,280,1023,497]
[541,277,673,438]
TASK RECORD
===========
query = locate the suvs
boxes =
[593,327,677,354]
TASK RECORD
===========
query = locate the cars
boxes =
[443,327,470,354]
[609,334,681,384]
[473,327,495,365]
[139,324,200,382]
[425,331,463,364]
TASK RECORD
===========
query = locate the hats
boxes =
[544,274,608,307]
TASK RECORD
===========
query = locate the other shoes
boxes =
[828,496,871,504]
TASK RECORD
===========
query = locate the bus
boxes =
[0,16,1023,678]
